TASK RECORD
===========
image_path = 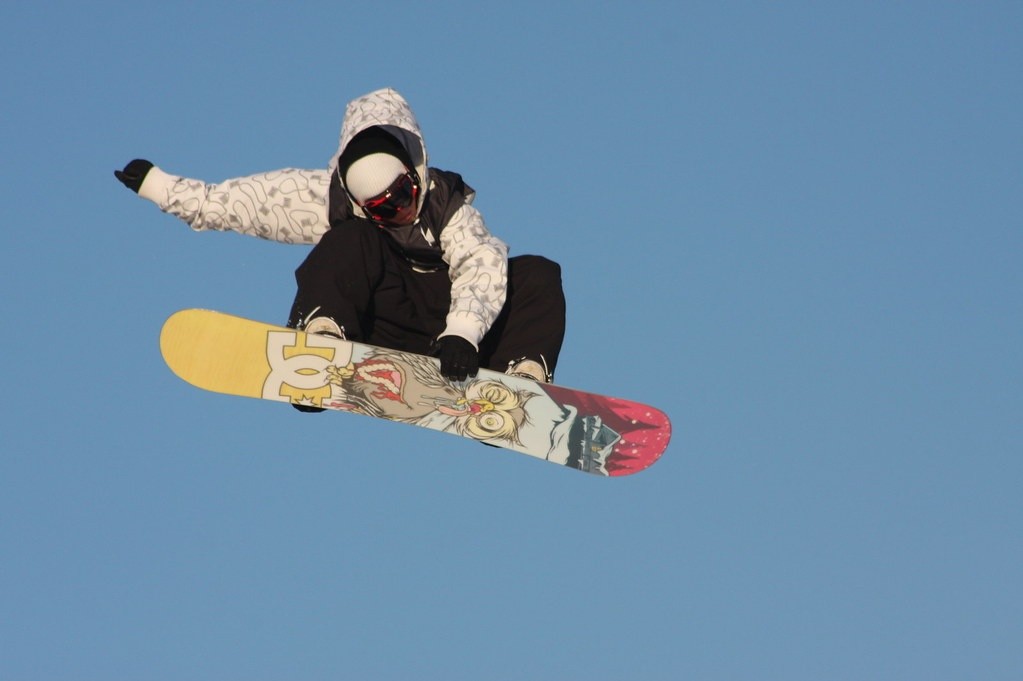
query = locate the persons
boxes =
[107,86,568,415]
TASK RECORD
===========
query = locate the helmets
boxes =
[346,153,408,207]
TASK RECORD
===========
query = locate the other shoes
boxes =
[509,359,546,383]
[304,318,342,338]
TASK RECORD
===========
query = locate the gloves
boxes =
[114,159,152,193]
[425,335,478,382]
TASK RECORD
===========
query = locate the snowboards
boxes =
[158,307,673,479]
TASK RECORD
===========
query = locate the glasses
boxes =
[366,176,414,220]
